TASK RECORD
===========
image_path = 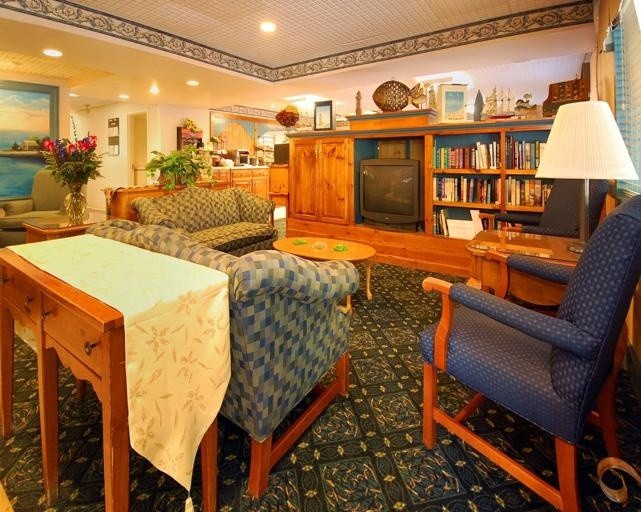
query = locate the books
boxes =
[426,137,554,238]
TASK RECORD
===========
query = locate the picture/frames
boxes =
[314,100,334,131]
[0,79,60,200]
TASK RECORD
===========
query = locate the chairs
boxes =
[0,169,85,247]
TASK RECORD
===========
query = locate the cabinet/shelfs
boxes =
[161,168,270,200]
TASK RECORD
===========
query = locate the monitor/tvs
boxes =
[359,158,422,232]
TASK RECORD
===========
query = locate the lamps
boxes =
[534,99,641,255]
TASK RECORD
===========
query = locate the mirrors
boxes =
[213,114,289,168]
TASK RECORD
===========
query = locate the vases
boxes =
[63,182,89,227]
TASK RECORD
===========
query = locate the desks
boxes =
[22,213,123,244]
[0,234,229,511]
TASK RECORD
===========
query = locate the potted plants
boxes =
[145,146,214,192]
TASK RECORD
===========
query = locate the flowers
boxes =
[37,114,102,194]
